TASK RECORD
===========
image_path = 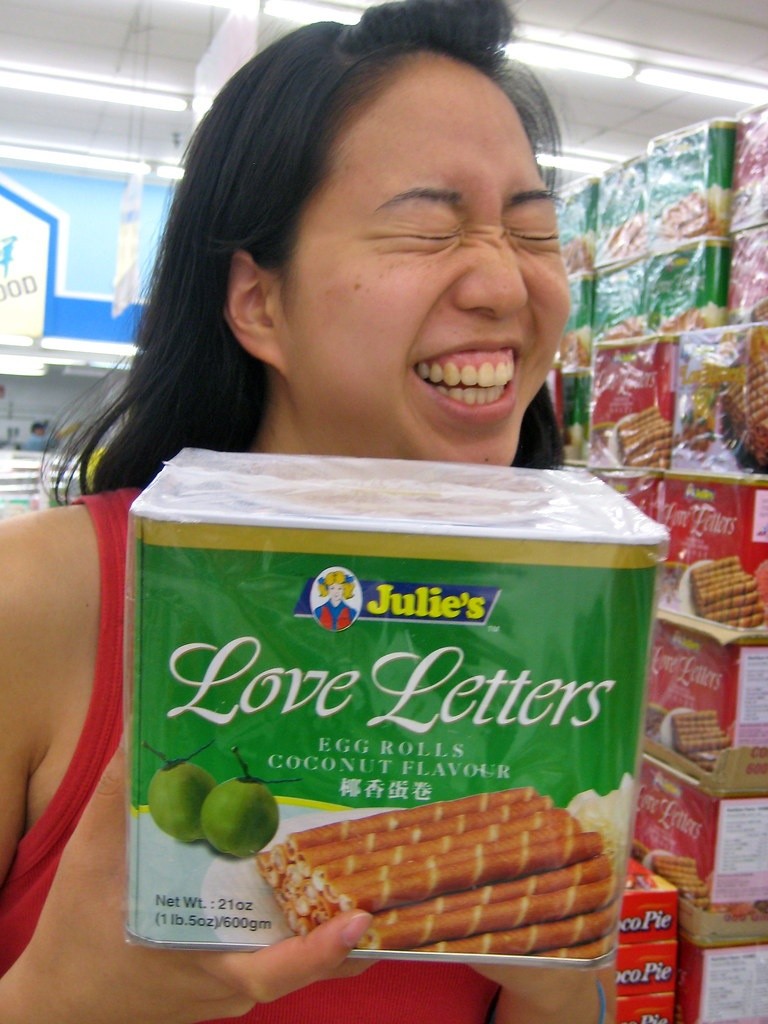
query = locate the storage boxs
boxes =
[122,106,767,1024]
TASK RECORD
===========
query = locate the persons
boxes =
[0,0,619,1024]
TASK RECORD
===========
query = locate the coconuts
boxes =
[149,763,280,858]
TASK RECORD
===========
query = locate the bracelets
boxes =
[491,979,607,1024]
